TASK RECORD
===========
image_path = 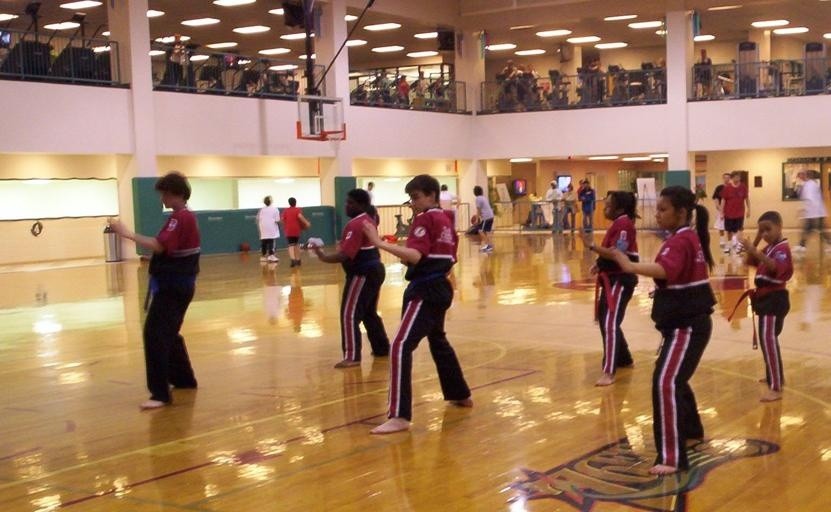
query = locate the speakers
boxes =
[280,0,305,27]
[438,30,454,50]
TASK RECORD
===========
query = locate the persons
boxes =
[107,172,201,409]
[795,255,825,330]
[580,234,596,279]
[694,48,712,101]
[546,180,564,235]
[365,182,374,205]
[744,402,785,511]
[563,185,578,233]
[313,189,390,367]
[396,75,411,108]
[256,195,281,262]
[368,406,474,512]
[476,252,495,312]
[582,190,640,386]
[494,60,518,82]
[261,262,283,326]
[713,251,728,303]
[719,254,748,331]
[281,197,311,269]
[285,269,311,333]
[363,174,474,435]
[720,171,751,254]
[524,65,540,92]
[474,186,495,252]
[656,57,667,104]
[657,476,692,511]
[528,235,547,272]
[340,367,390,475]
[413,71,431,97]
[580,182,596,234]
[552,235,563,265]
[587,58,607,105]
[713,173,739,249]
[566,234,578,262]
[142,409,207,511]
[738,211,794,401]
[595,387,636,483]
[526,192,545,227]
[439,185,463,211]
[791,170,830,254]
[157,34,185,89]
[505,64,524,81]
[614,184,718,475]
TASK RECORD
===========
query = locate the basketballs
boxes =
[240,243,248,250]
[472,216,479,224]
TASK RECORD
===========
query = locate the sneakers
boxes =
[481,244,492,251]
[267,255,278,262]
[296,259,301,264]
[259,256,267,261]
[291,260,296,267]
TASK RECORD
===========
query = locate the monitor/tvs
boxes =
[511,179,527,195]
[0,28,12,48]
[557,175,572,193]
